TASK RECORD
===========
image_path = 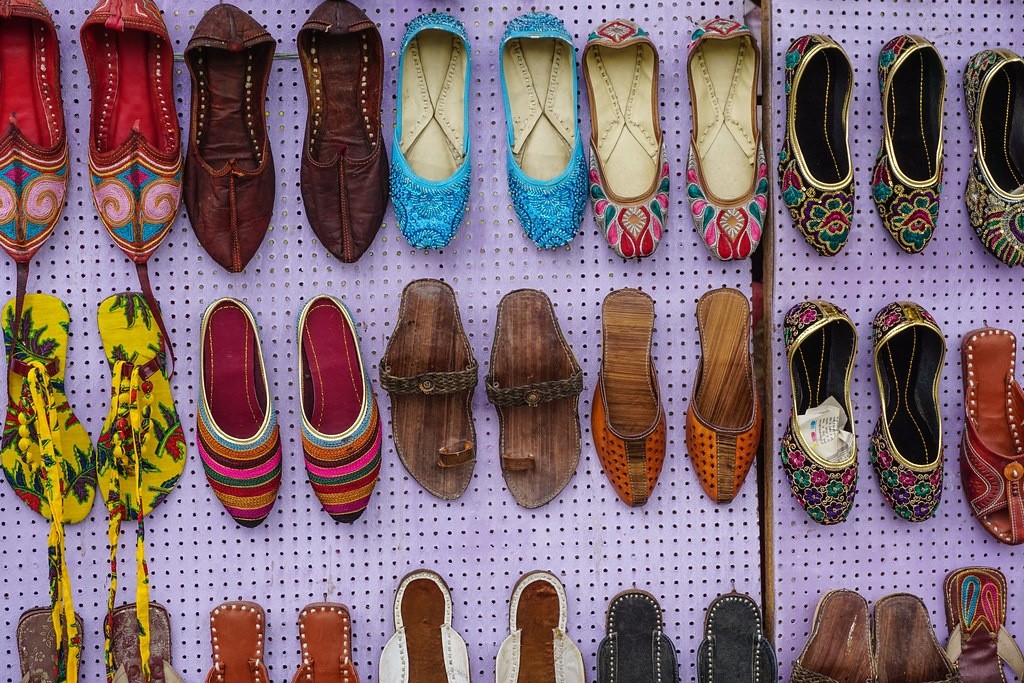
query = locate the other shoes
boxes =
[963,47,1023,265]
[870,302,947,523]
[781,301,859,527]
[1,0,68,261]
[500,14,590,248]
[184,3,275,273]
[687,18,770,261]
[389,14,468,250]
[297,294,381,522]
[871,35,946,254]
[78,0,184,264]
[298,0,388,264]
[778,35,857,256]
[583,19,670,258]
[193,297,281,529]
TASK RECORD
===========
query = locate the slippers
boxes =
[381,280,478,500]
[961,327,1023,546]
[591,287,667,507]
[687,289,759,505]
[95,293,187,682]
[486,290,584,511]
[0,292,91,683]
[17,569,1024,683]
